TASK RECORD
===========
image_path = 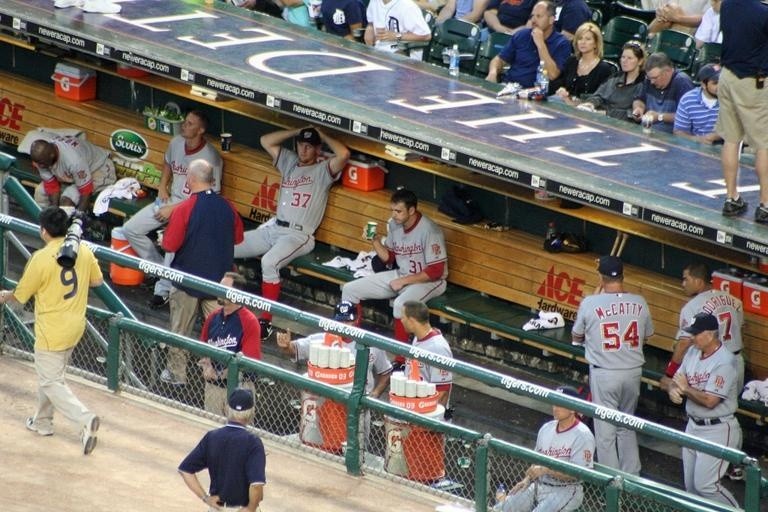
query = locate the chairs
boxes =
[588,8,724,83]
[419,8,514,83]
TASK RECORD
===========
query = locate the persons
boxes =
[27,131,115,226]
[120,113,223,311]
[342,188,451,369]
[0,208,106,456]
[398,301,455,440]
[213,124,351,341]
[715,0,768,224]
[198,271,256,423]
[209,0,722,153]
[665,312,745,510]
[179,389,266,511]
[275,302,394,451]
[660,262,744,482]
[52,0,125,16]
[489,386,596,511]
[572,255,657,507]
[159,158,244,383]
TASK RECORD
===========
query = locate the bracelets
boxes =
[666,359,680,377]
[203,493,210,503]
[2,291,5,301]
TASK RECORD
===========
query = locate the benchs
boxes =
[0,140,768,427]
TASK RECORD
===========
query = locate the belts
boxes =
[216,500,244,508]
[689,416,720,426]
[274,218,303,230]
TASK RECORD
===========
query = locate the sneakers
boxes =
[148,294,169,310]
[139,277,160,290]
[25,416,55,436]
[81,414,101,457]
[724,464,744,480]
[259,317,275,342]
[755,203,768,223]
[723,197,749,217]
[161,370,187,384]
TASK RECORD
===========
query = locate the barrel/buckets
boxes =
[298,373,356,456]
[109,227,145,285]
[383,404,446,483]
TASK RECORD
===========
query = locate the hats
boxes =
[555,385,581,398]
[334,301,356,321]
[598,256,624,277]
[696,61,721,83]
[296,128,321,147]
[681,312,719,335]
[227,388,254,411]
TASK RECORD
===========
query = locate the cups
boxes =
[155,230,165,250]
[390,369,437,396]
[375,25,384,38]
[641,115,654,134]
[220,133,231,152]
[366,221,377,240]
[308,339,354,368]
[308,0,322,20]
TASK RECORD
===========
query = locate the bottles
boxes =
[546,222,558,243]
[536,61,549,93]
[449,45,459,77]
[495,485,506,505]
